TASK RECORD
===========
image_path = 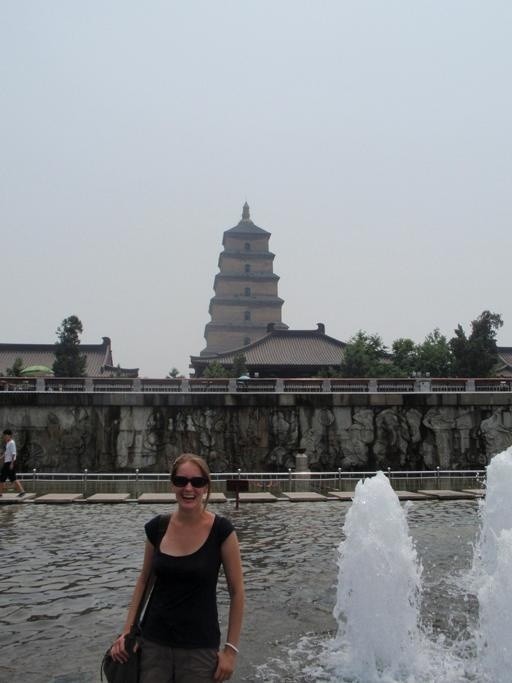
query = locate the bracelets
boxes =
[225,642,239,654]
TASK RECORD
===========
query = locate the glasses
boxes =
[172,476,207,487]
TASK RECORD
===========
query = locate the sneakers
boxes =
[17,492,26,497]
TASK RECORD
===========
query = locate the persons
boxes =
[108,451,245,682]
[1,428,27,498]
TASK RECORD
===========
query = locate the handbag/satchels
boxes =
[103,626,139,683]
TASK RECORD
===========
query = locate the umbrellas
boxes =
[19,364,55,376]
